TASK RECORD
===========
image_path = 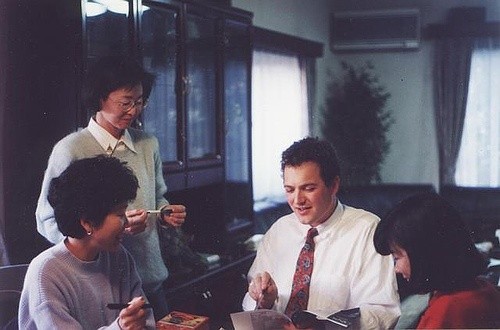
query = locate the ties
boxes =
[283,227,319,321]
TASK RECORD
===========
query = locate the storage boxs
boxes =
[156,311,209,330]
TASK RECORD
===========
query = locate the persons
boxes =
[373,193,500,330]
[242,136,403,330]
[36,56,187,321]
[18,154,155,330]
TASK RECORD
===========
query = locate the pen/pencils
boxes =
[146,209,172,214]
[106,303,153,310]
[254,282,271,309]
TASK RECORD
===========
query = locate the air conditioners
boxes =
[331,8,422,50]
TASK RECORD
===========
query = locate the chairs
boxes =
[0,264,31,330]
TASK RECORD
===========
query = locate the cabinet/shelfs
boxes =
[0,0,255,264]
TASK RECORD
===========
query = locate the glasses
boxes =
[107,95,148,113]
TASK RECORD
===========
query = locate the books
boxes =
[230,308,360,330]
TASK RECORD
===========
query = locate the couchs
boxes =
[236,185,500,246]
